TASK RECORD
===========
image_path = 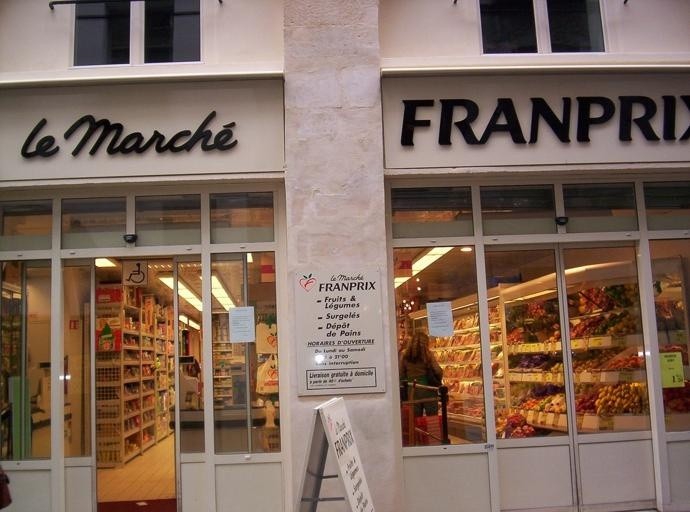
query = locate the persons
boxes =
[397,331,444,416]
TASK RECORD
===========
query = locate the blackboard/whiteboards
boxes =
[294,397,373,512]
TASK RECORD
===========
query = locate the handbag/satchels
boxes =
[427,366,443,387]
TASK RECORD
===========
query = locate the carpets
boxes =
[97,498,177,512]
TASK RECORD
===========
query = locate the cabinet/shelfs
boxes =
[500,272,569,436]
[0,283,28,460]
[563,262,651,434]
[650,255,690,431]
[82,301,174,468]
[212,311,233,409]
[407,284,505,442]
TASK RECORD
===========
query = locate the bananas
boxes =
[596,383,649,417]
